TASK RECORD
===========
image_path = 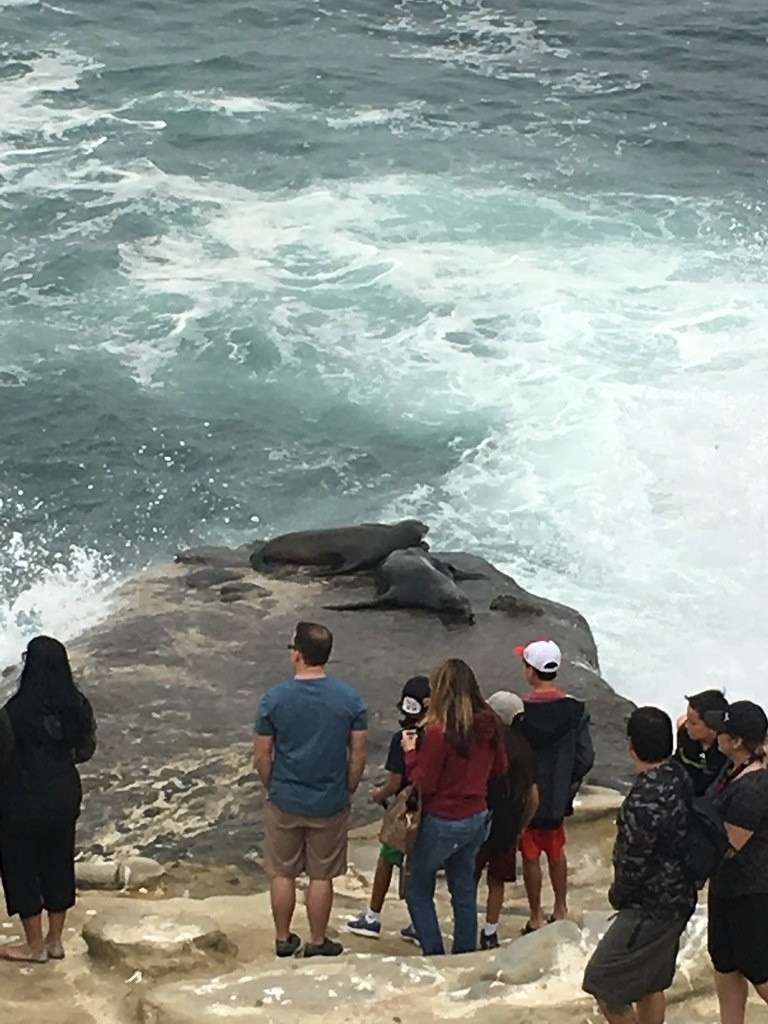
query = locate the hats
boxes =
[703,701,768,743]
[396,675,431,715]
[485,689,526,726]
[511,637,562,672]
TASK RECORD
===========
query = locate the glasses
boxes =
[287,644,297,650]
[22,651,28,661]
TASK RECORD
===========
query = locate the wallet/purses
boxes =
[485,809,493,823]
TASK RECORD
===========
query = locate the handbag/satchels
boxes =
[685,803,730,879]
[378,781,422,855]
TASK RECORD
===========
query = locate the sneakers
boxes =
[275,934,300,957]
[293,937,343,958]
[547,913,556,923]
[480,928,501,951]
[399,923,421,946]
[345,913,381,938]
[521,921,537,935]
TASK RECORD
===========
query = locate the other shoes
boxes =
[0,940,65,963]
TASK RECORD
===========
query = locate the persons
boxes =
[347,638,768,1024]
[0,635,97,963]
[253,620,368,958]
